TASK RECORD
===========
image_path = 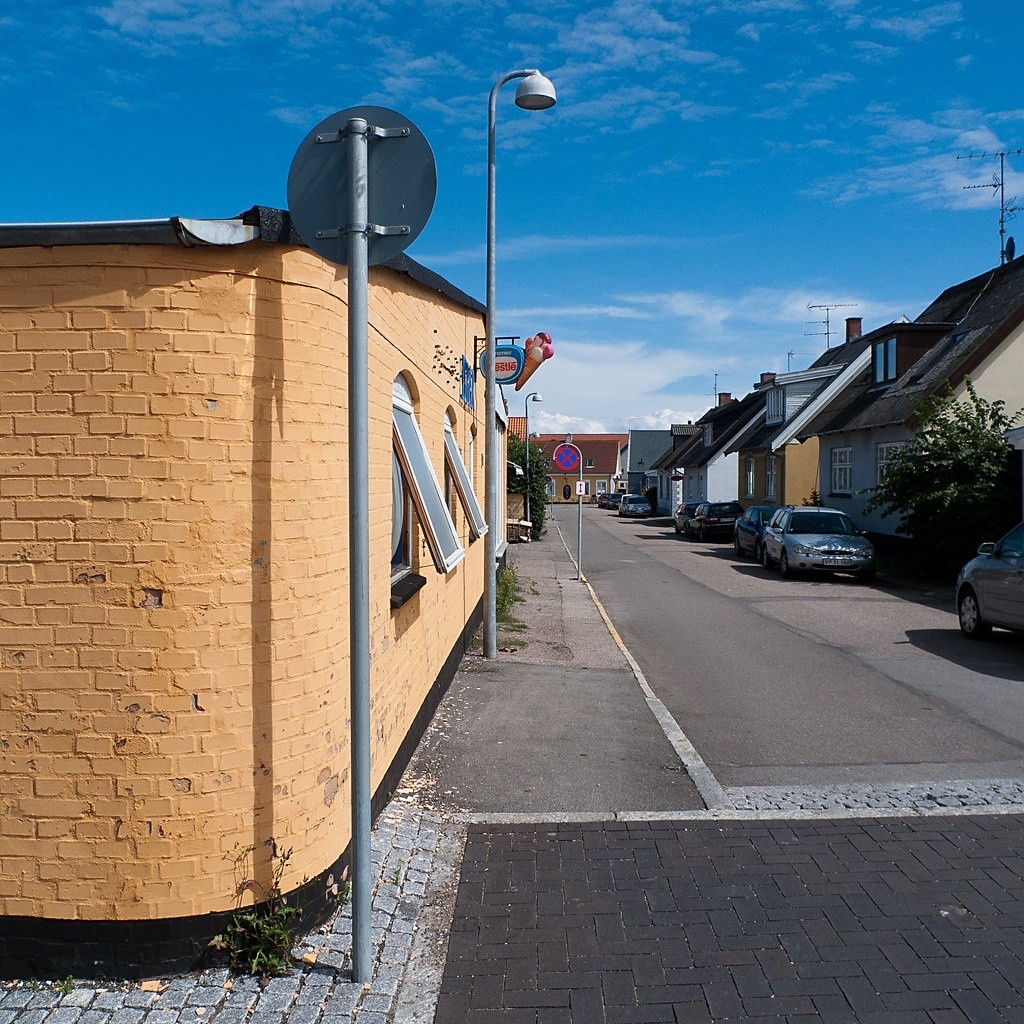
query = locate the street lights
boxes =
[483,69,556,659]
[525,393,543,542]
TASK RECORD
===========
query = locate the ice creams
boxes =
[515,332,554,391]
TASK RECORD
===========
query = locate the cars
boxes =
[956,521,1024,640]
[762,505,875,580]
[618,494,651,517]
[598,493,624,510]
[734,506,780,563]
[674,501,745,542]
